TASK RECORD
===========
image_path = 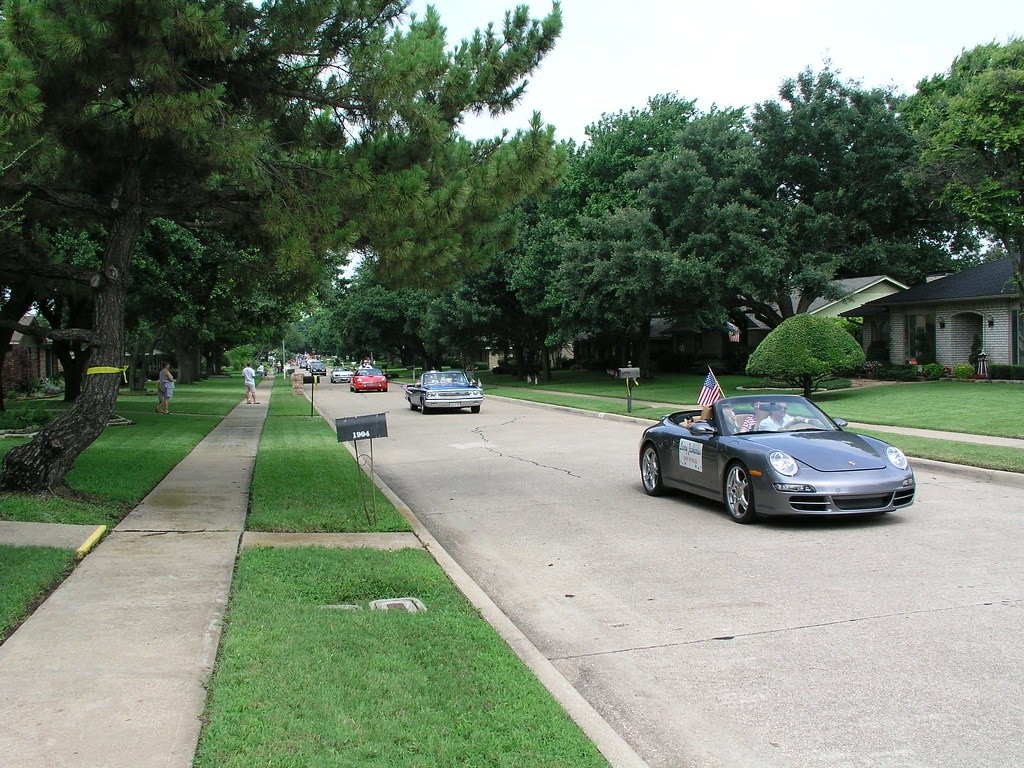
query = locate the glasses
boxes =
[780,406,787,410]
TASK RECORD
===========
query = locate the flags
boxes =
[726,322,740,342]
[697,373,720,409]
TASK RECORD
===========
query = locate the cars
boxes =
[330,366,354,384]
[350,369,388,393]
[298,357,326,376]
[638,395,915,524]
[405,372,484,415]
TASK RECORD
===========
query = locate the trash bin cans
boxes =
[288,369,295,375]
[326,359,335,367]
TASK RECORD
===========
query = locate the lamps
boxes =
[938,317,946,329]
[986,315,994,327]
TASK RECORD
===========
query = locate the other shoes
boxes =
[254,401,260,404]
[156,407,160,413]
[164,412,173,415]
[247,402,252,404]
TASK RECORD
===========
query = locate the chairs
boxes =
[700,405,771,431]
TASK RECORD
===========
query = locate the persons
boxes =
[740,402,760,433]
[155,362,177,414]
[277,360,281,373]
[256,363,263,372]
[758,401,805,432]
[241,363,261,404]
